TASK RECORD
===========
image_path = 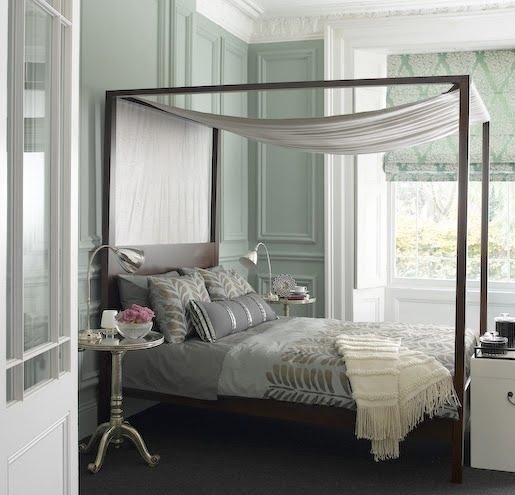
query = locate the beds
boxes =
[107,240,487,488]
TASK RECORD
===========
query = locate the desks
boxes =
[262,295,317,316]
[73,329,165,472]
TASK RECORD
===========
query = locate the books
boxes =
[288,293,310,301]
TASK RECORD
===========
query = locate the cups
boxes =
[294,286,308,293]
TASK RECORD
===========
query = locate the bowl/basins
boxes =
[115,322,153,343]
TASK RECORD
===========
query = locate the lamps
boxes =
[78,244,144,339]
[240,241,278,300]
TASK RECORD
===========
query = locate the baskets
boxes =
[495,321,515,348]
[480,341,507,357]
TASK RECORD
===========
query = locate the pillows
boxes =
[114,262,278,342]
